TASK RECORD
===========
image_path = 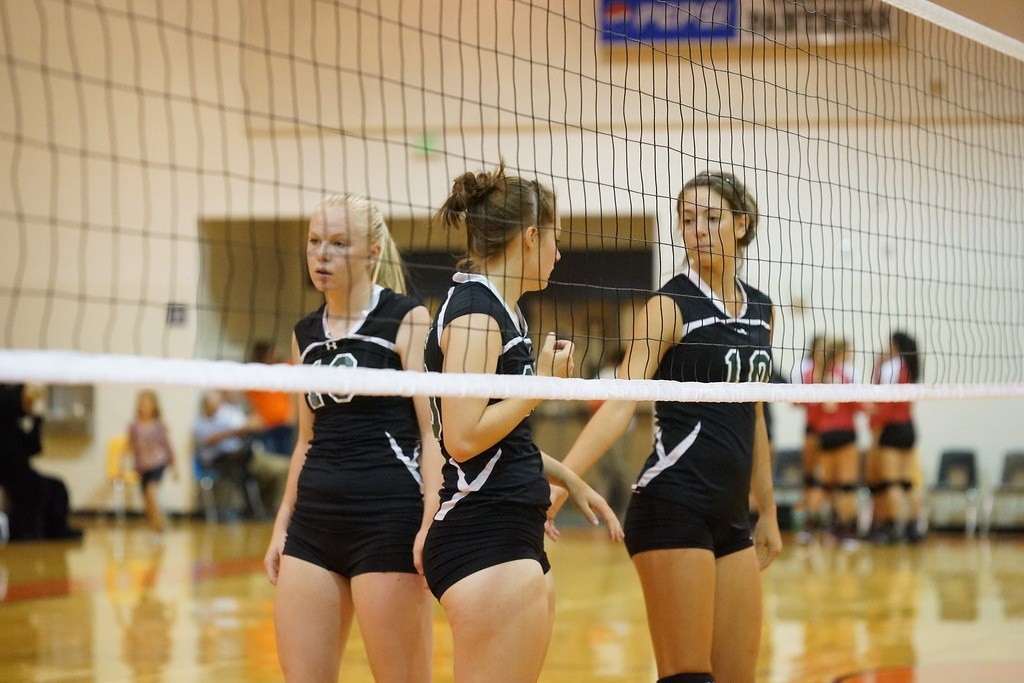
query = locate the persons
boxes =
[585,344,636,533]
[187,341,299,523]
[4,395,83,538]
[120,387,182,530]
[540,171,787,682]
[794,334,940,552]
[421,166,629,683]
[262,194,448,683]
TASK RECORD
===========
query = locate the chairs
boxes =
[771,448,810,534]
[921,448,981,540]
[89,435,148,525]
[980,449,1024,538]
[191,451,224,525]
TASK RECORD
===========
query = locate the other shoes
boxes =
[803,512,929,547]
